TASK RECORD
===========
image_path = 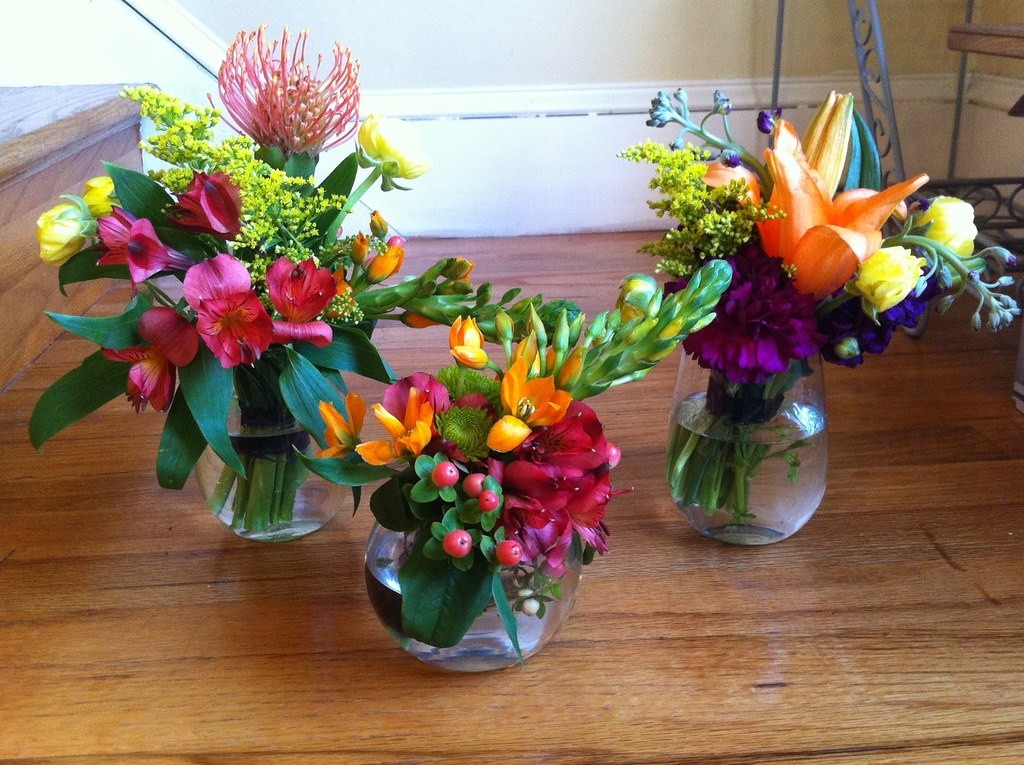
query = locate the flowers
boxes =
[612,87,1021,520]
[292,260,734,669]
[30,22,581,531]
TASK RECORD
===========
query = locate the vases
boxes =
[366,510,586,673]
[664,343,829,548]
[189,345,350,542]
